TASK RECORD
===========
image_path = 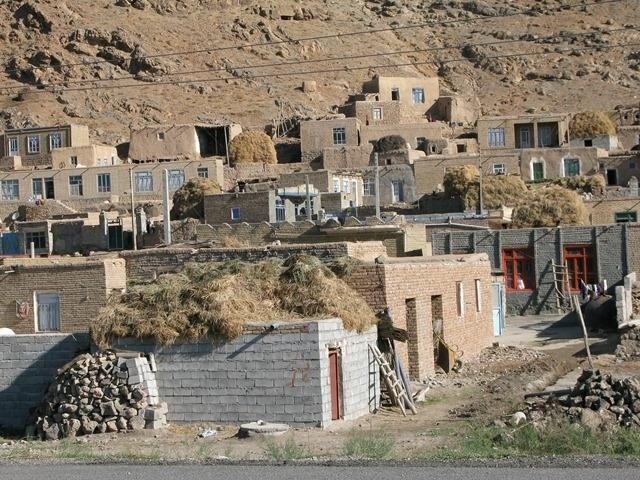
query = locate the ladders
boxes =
[551,258,574,315]
[368,344,418,417]
